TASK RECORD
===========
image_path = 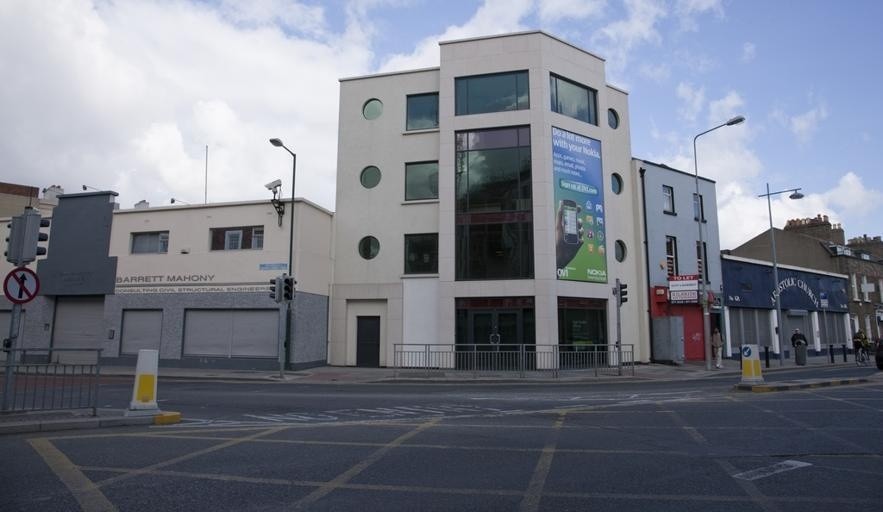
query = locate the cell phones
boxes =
[562,199,579,245]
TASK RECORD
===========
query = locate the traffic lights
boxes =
[282,274,294,303]
[21,206,50,267]
[615,282,628,308]
[3,214,24,264]
[267,277,282,305]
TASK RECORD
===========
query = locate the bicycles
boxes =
[852,337,870,368]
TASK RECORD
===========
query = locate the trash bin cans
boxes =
[794,338,806,365]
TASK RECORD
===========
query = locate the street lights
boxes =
[690,112,745,371]
[757,180,804,368]
[268,134,297,369]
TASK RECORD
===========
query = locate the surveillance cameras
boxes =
[264,179,282,190]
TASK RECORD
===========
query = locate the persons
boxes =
[853,328,865,361]
[709,326,724,369]
[555,199,585,280]
[790,329,809,361]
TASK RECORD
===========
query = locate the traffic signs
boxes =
[667,274,699,291]
[667,288,701,304]
